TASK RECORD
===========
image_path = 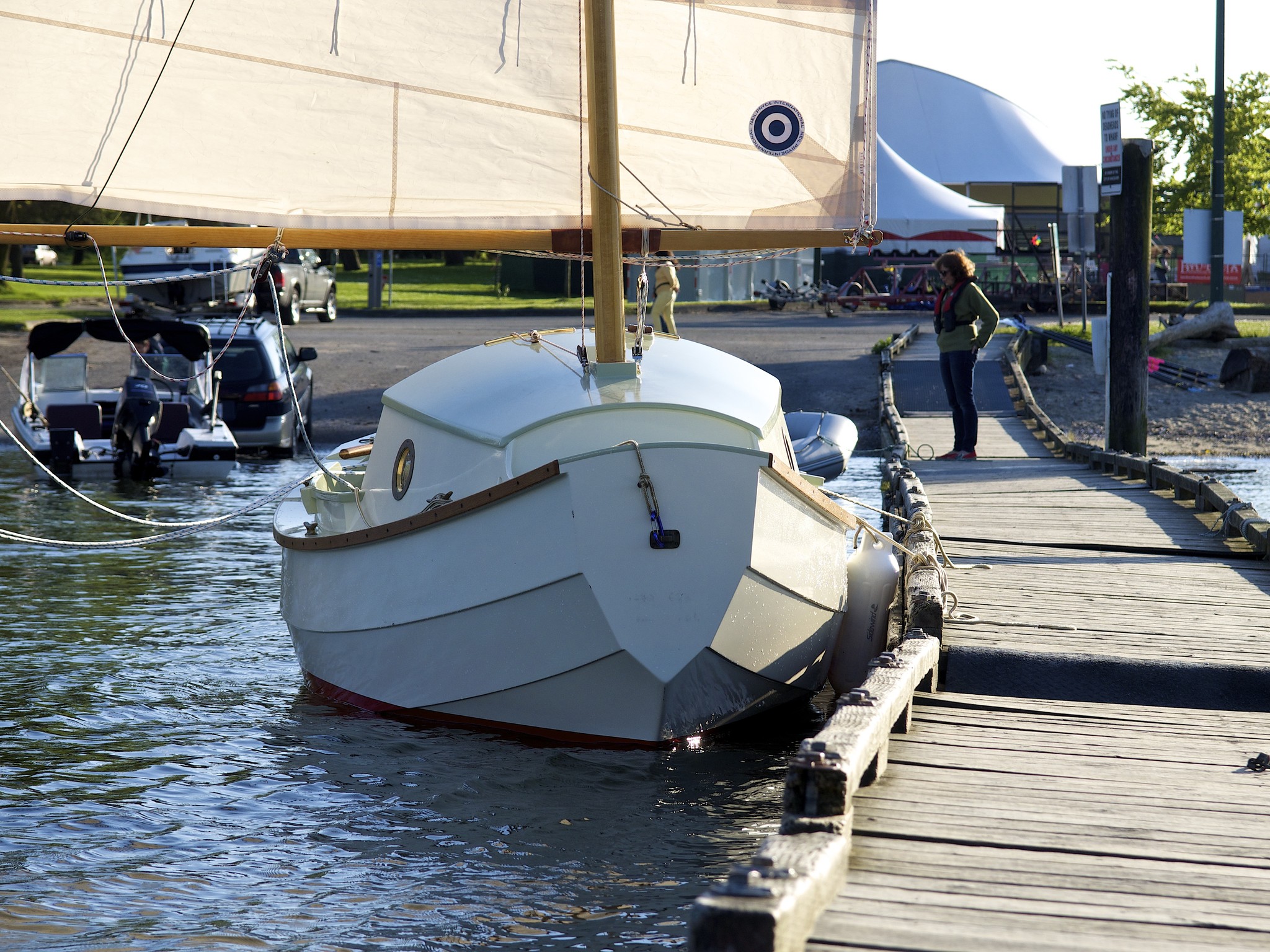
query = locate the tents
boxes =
[821,131,1006,307]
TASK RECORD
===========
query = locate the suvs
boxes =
[132,316,315,460]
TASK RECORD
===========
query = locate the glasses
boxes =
[938,270,950,277]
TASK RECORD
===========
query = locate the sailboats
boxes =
[0,0,901,751]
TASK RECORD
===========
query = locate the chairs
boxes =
[152,400,193,444]
[47,403,104,440]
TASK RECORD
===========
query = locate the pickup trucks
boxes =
[250,247,339,323]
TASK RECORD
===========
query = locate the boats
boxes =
[12,319,239,481]
[122,217,271,315]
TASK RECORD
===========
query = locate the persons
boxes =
[1149,246,1171,301]
[651,250,681,337]
[933,248,999,463]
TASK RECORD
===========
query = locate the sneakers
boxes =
[946,449,977,462]
[935,450,962,461]
